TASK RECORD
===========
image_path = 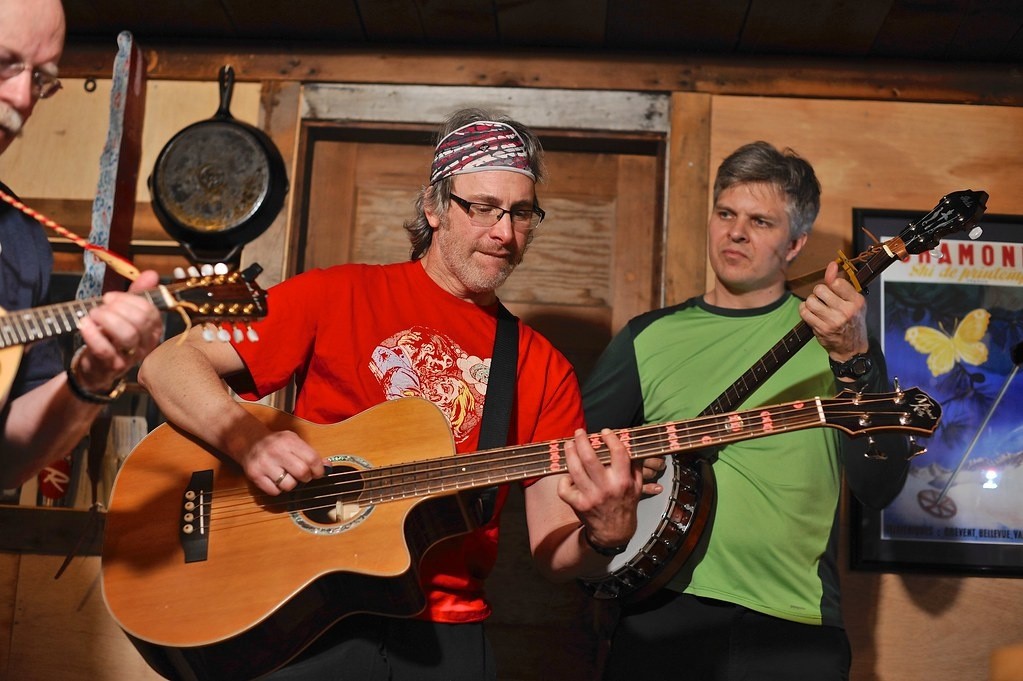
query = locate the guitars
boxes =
[98,376,944,679]
[0,261,270,352]
[589,188,990,603]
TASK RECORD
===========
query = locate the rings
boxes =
[123,347,137,355]
[275,471,287,487]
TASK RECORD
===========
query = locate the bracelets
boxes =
[68,345,126,404]
[585,529,629,556]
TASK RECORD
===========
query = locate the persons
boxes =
[579,140,908,680]
[0,0,164,508]
[138,109,642,680]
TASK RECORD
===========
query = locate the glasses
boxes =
[448,192,546,230]
[0,47,63,98]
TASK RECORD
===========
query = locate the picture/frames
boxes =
[851,206,1023,579]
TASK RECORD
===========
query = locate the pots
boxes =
[146,66,291,264]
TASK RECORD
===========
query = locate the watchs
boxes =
[829,347,873,380]
